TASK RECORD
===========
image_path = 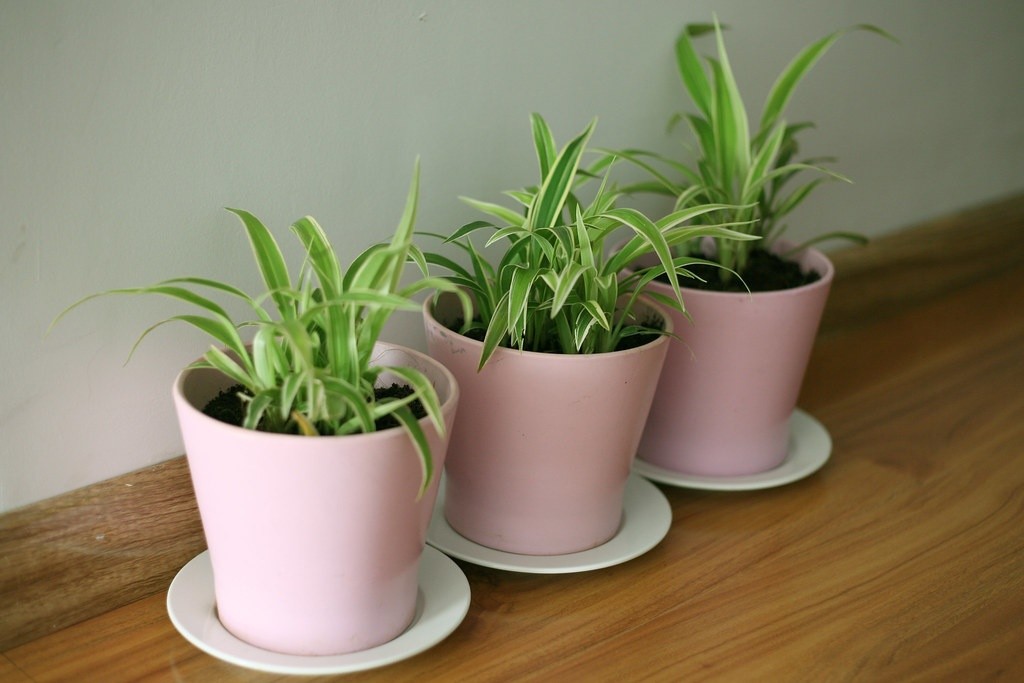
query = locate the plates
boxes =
[168,538,473,674]
[425,467,673,575]
[629,406,832,491]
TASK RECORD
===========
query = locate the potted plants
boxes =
[45,16,908,655]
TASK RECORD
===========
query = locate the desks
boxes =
[0,195,1024,683]
